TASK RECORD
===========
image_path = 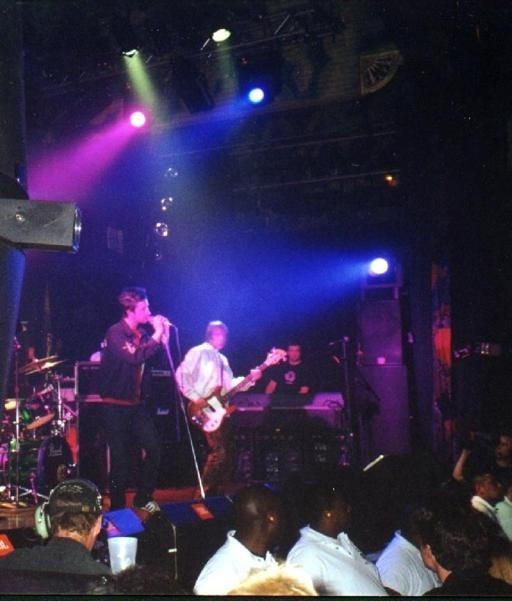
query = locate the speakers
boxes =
[74,360,181,488]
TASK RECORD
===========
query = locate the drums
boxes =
[4,436,73,501]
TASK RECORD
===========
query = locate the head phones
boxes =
[33,479,102,542]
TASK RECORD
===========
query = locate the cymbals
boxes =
[15,356,69,374]
[26,414,55,430]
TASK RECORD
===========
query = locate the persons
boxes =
[100,285,171,512]
[267,338,314,396]
[25,305,66,383]
[175,320,263,501]
[3,423,510,599]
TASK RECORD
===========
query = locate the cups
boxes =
[107,535,137,574]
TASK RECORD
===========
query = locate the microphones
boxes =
[148,315,174,328]
[331,336,350,348]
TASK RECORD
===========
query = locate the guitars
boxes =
[187,347,287,433]
